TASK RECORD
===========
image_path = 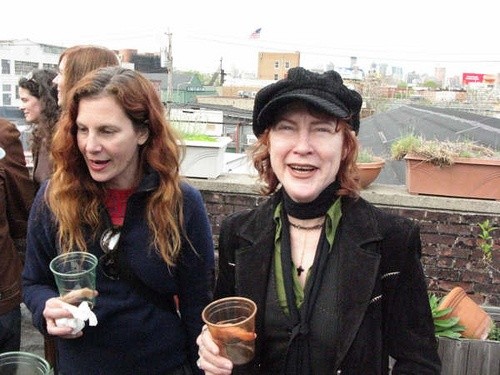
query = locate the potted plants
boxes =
[170,118,233,179]
[390,129,500,200]
[436,322,500,375]
[356,148,386,188]
[428,287,494,340]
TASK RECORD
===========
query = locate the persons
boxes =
[19,70,66,186]
[52,44,120,107]
[196,68,442,375]
[289,222,325,276]
[0,117,37,375]
[22,66,216,375]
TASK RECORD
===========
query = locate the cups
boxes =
[0,351,51,375]
[49,252,98,310]
[202,296,258,365]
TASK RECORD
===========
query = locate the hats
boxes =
[252,67,363,142]
[18,69,58,102]
